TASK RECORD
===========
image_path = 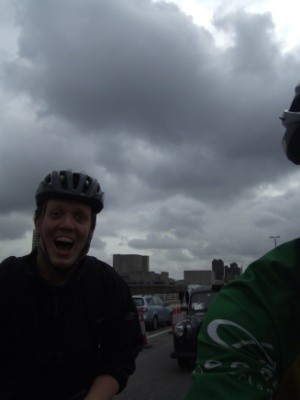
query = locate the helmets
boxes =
[35,170,103,215]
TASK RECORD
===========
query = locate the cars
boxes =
[173,283,218,371]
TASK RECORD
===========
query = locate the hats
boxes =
[279,83,300,166]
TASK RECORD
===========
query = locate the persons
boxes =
[191,88,300,400]
[0,168,143,400]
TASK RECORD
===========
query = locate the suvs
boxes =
[132,294,174,331]
